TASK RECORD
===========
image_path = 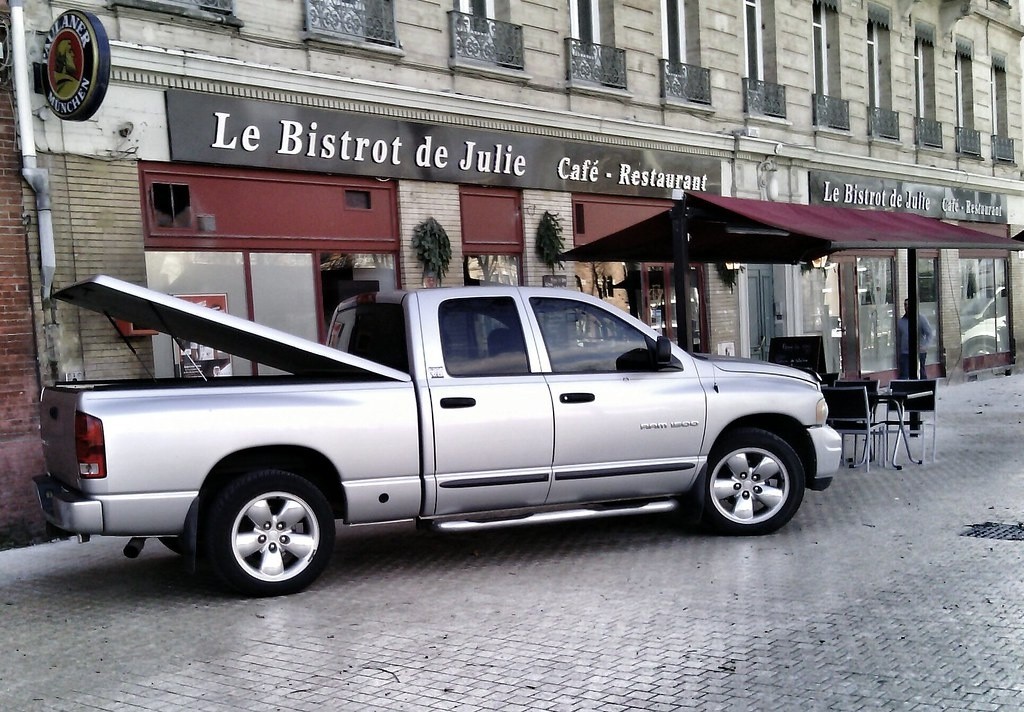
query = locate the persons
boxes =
[348,334,385,364]
[896,300,931,379]
[443,329,526,376]
[487,329,635,372]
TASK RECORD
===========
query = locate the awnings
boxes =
[682,190,1024,263]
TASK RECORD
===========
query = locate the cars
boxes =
[959,286,1007,357]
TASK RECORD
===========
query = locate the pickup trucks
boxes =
[30,272,846,597]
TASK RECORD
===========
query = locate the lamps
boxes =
[813,255,828,268]
[725,263,741,270]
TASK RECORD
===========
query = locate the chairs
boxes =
[821,377,938,473]
[487,327,527,372]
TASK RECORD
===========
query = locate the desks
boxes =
[849,390,933,470]
